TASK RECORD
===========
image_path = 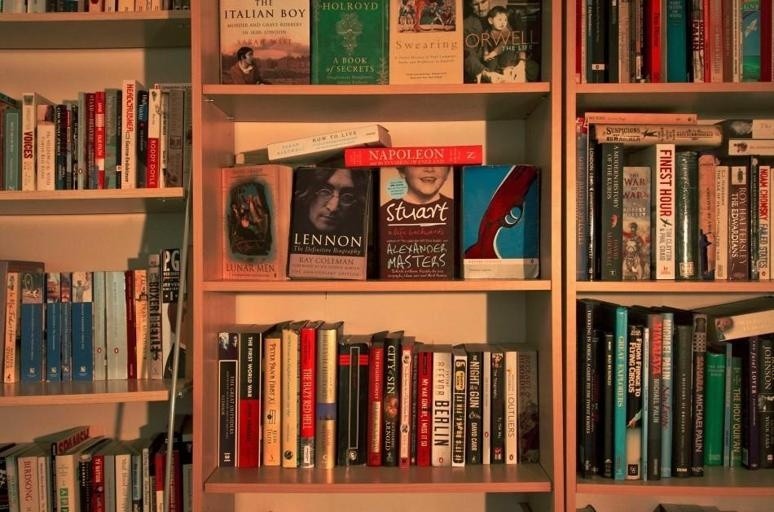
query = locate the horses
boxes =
[621,236,644,281]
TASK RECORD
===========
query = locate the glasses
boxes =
[313,187,358,208]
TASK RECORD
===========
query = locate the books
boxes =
[2,0,190,12]
[576,298,774,511]
[0,80,191,192]
[286,161,374,281]
[219,163,294,283]
[308,2,391,85]
[455,159,543,282]
[387,1,464,86]
[1,414,194,512]
[572,107,774,281]
[266,125,393,165]
[575,1,774,85]
[341,143,486,170]
[209,315,542,474]
[0,244,195,383]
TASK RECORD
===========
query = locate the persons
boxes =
[229,43,269,85]
[293,166,367,257]
[482,6,519,75]
[218,0,309,86]
